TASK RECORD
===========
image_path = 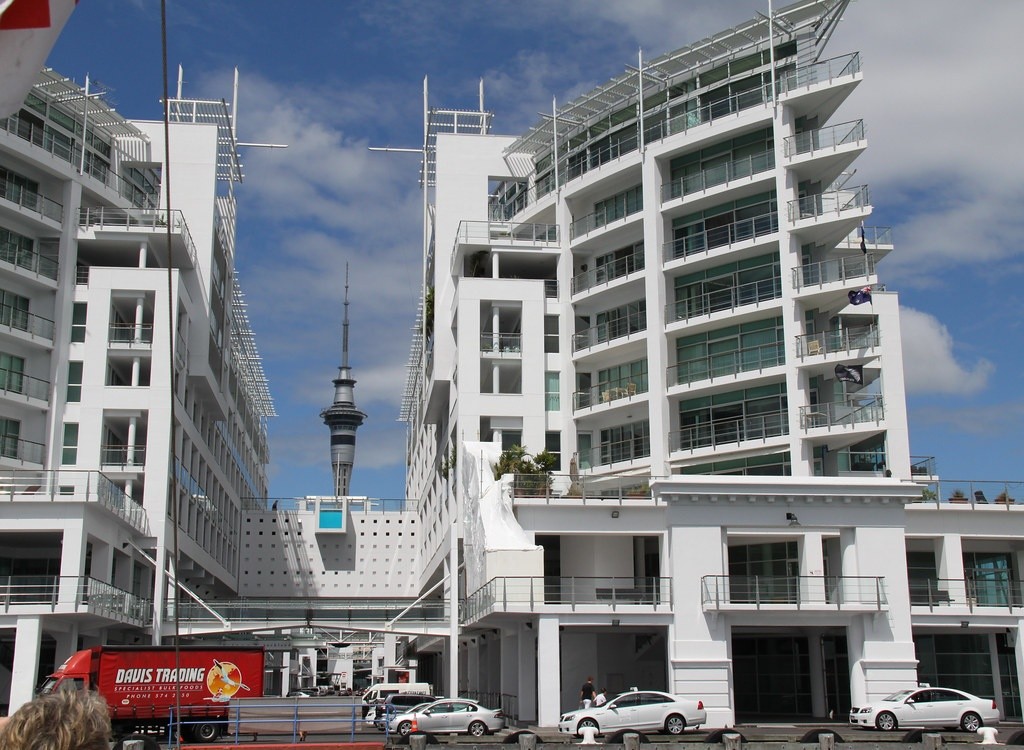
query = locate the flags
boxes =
[848,285,873,305]
[835,363,864,386]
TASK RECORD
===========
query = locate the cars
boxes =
[850,681,1001,733]
[287,675,506,740]
[559,688,706,737]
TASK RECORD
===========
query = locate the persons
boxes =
[0,682,113,750]
[577,676,597,710]
[592,687,608,707]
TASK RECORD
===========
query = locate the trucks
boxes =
[35,640,264,741]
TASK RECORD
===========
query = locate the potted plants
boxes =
[996,493,1014,504]
[854,458,874,471]
[948,489,968,503]
[495,445,555,495]
